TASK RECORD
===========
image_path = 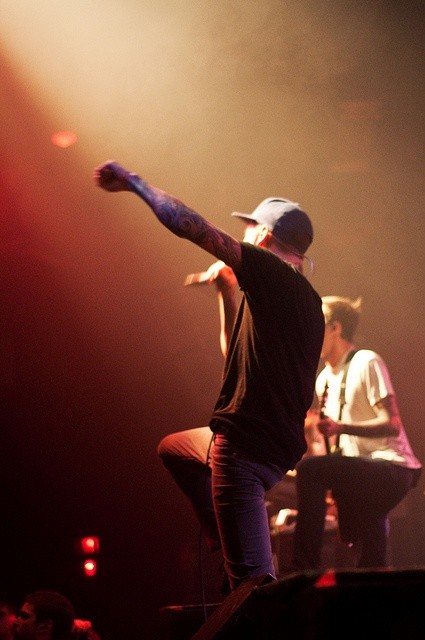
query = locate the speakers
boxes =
[193,566,425,640]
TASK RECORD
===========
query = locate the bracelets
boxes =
[343,424,348,434]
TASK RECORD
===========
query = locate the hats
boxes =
[231,196,313,255]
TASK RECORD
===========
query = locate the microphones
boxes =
[184,272,208,287]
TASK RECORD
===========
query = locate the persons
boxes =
[96,161,324,594]
[0,592,98,640]
[288,295,422,572]
